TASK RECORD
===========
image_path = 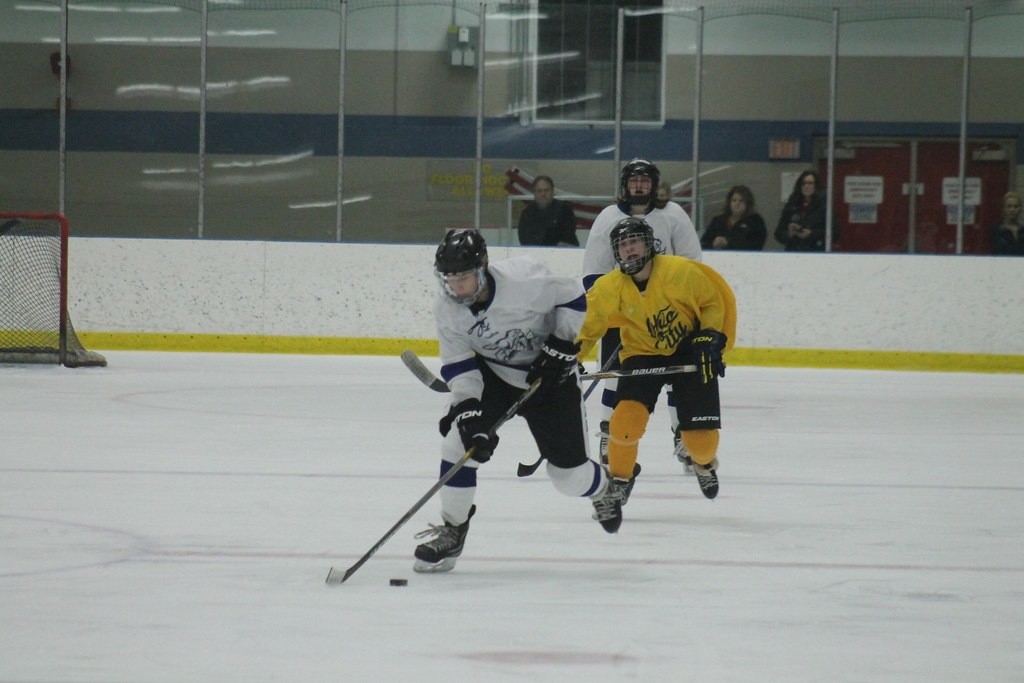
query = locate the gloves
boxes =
[691,329,727,385]
[525,333,582,400]
[455,398,499,463]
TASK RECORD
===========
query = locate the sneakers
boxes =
[592,465,622,533]
[415,505,476,573]
[671,423,693,472]
[691,458,718,499]
[600,420,609,464]
[608,463,641,506]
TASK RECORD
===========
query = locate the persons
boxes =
[772,171,841,253]
[410,228,624,576]
[570,158,739,504]
[700,184,768,252]
[517,175,580,248]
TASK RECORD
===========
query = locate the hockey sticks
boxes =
[514,340,625,479]
[324,374,543,589]
[398,347,727,394]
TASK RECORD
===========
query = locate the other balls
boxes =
[388,579,408,587]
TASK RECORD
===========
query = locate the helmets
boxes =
[620,159,660,205]
[610,218,655,276]
[433,229,488,309]
